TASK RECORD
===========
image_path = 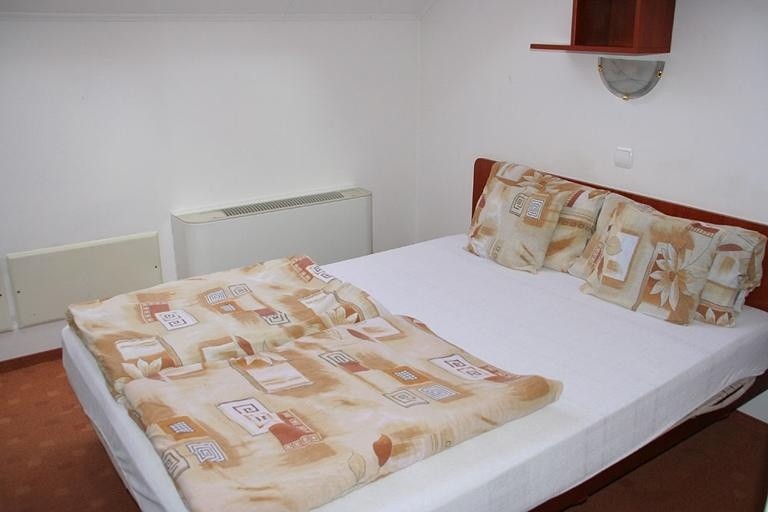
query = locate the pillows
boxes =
[469,161,610,283]
[577,196,765,326]
[574,198,726,328]
[466,176,577,277]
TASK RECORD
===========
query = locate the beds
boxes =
[59,154,767,512]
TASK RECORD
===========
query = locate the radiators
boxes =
[173,188,375,278]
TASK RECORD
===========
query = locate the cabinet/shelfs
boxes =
[526,1,681,55]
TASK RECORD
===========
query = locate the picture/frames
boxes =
[599,56,668,107]
